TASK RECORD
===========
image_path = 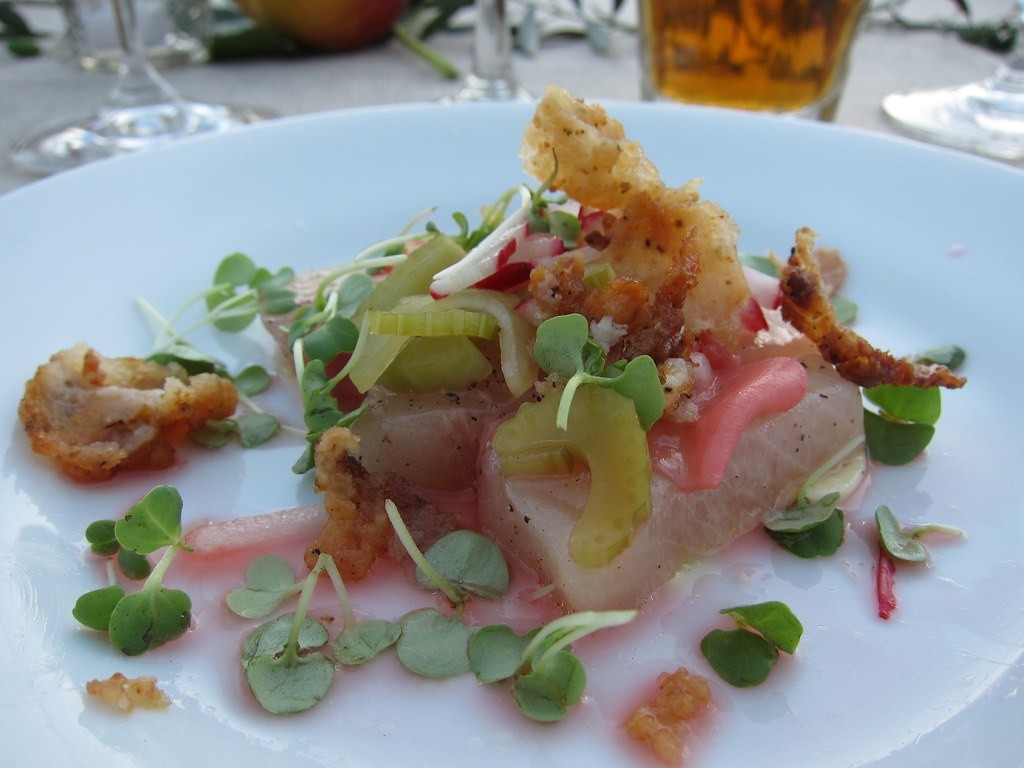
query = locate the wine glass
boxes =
[441,0,545,104]
[8,0,285,176]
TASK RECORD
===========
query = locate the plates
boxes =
[0,96,1024,768]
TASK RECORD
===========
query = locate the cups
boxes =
[64,0,216,73]
[639,0,870,123]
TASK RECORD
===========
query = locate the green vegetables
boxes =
[73,145,969,723]
